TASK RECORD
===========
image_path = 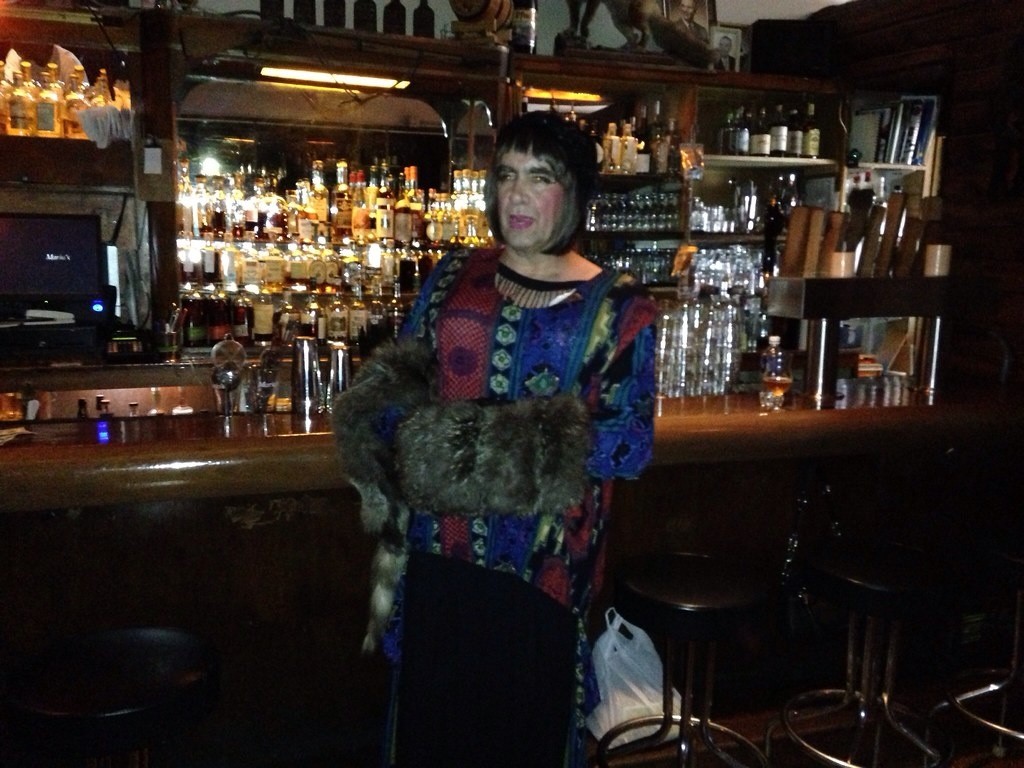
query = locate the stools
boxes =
[949,559,1024,758]
[597,548,772,768]
[0,624,221,768]
[765,542,956,768]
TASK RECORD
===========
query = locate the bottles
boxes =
[354,0,377,32]
[76,399,88,418]
[759,335,780,402]
[512,0,538,55]
[0,60,133,139]
[586,99,681,284]
[101,400,113,418]
[323,0,346,28]
[737,173,800,232]
[413,0,434,38]
[260,0,284,21]
[172,386,194,414]
[178,151,492,352]
[95,394,104,418]
[384,0,405,35]
[293,0,317,24]
[147,387,167,416]
[722,101,821,158]
[129,402,140,416]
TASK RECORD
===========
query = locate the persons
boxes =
[713,36,736,70]
[675,0,707,39]
[332,110,659,768]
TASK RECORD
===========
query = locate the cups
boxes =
[155,332,178,363]
[688,198,766,294]
[212,384,241,416]
[292,336,320,415]
[655,298,740,398]
[241,364,278,412]
[326,349,349,414]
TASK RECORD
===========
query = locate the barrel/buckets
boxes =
[449,0,523,38]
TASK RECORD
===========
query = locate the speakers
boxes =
[744,20,846,83]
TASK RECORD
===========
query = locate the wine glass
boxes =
[763,351,793,413]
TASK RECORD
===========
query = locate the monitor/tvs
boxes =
[0,212,106,328]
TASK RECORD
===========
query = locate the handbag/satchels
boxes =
[585,607,682,750]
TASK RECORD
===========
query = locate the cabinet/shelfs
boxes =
[1,2,846,413]
